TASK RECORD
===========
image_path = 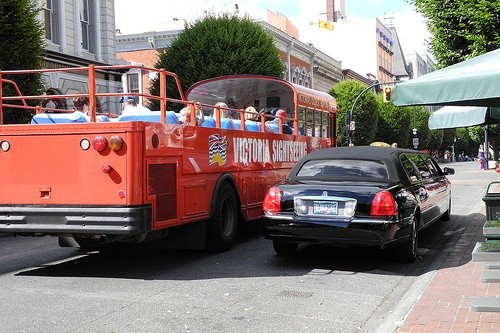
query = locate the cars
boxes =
[260,146,455,264]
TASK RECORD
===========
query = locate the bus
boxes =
[0,65,339,256]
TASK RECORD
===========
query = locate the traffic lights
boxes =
[383,86,393,103]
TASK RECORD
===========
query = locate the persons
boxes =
[32,88,295,135]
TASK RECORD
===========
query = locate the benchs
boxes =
[30,111,280,134]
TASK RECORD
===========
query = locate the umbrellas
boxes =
[391,47,500,130]
